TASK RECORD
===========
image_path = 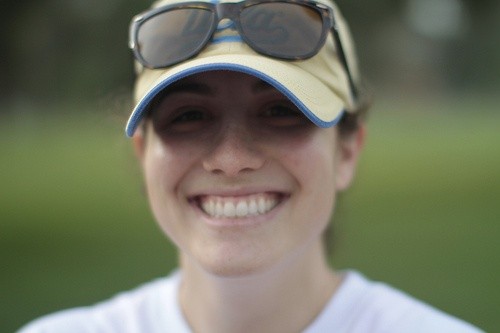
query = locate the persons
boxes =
[0,0,490,332]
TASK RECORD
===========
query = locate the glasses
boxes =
[128,0,359,99]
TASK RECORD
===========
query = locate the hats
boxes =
[125,1,360,139]
